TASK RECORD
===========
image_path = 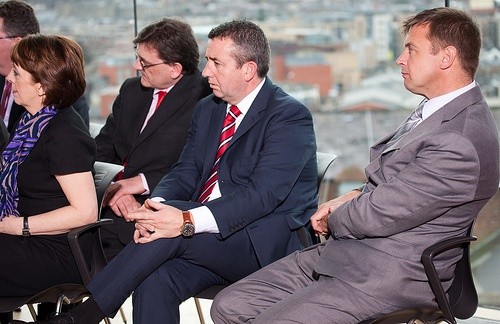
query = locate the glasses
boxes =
[135,52,168,71]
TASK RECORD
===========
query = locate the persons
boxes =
[37,18,214,323]
[48,20,320,324]
[210,8,500,324]
[0,34,100,314]
[0,0,91,141]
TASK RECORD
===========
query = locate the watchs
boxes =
[180,210,195,236]
[22,216,31,236]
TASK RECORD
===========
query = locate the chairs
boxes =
[0,124,479,324]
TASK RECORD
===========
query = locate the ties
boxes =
[0,81,12,119]
[382,101,427,152]
[196,105,242,204]
[117,91,168,180]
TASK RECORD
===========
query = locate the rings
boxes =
[147,230,155,234]
